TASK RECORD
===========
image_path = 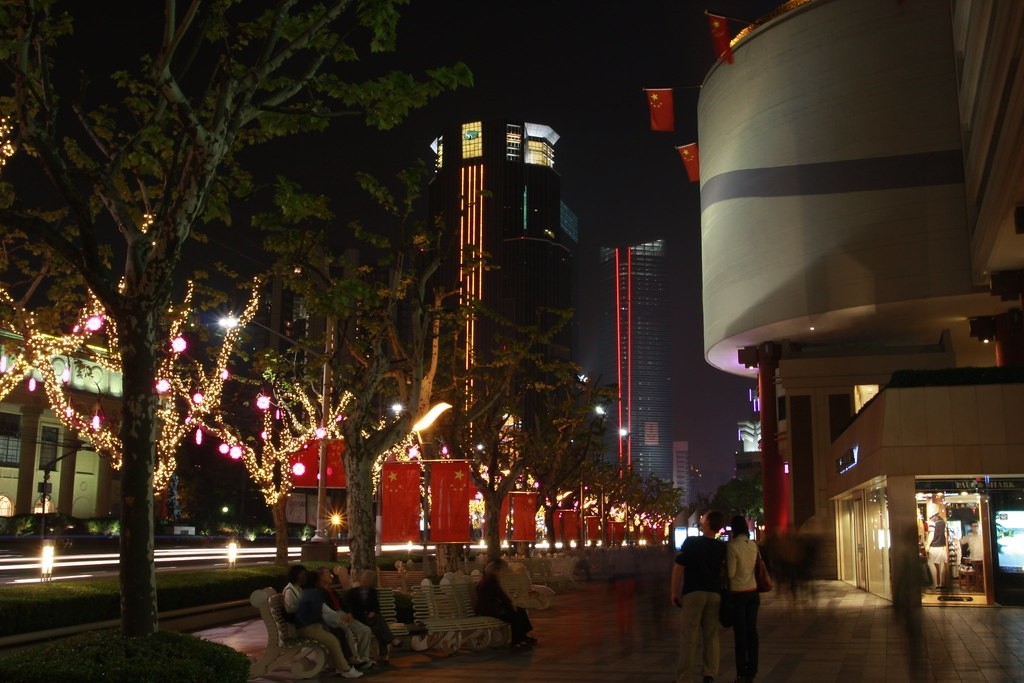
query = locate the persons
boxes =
[281,564,404,678]
[573,556,593,582]
[925,510,959,591]
[472,558,538,651]
[775,522,822,601]
[672,509,760,683]
[959,521,983,591]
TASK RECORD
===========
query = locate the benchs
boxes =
[411,583,511,656]
[336,588,410,659]
[396,559,422,570]
[443,570,481,585]
[334,566,362,590]
[246,587,332,677]
[376,565,425,594]
[476,544,665,610]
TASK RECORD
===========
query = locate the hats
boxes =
[485,561,502,573]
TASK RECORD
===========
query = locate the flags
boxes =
[287,437,666,545]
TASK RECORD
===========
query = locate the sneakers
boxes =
[335,668,341,674]
[341,666,363,678]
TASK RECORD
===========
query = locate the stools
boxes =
[959,569,978,591]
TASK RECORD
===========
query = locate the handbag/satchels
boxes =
[284,612,296,624]
[755,558,773,592]
[719,588,738,627]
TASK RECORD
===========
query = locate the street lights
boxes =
[225,310,338,563]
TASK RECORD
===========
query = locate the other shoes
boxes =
[362,661,376,669]
[732,676,746,683]
[703,675,714,683]
[392,639,404,648]
[525,637,538,643]
[350,661,368,668]
